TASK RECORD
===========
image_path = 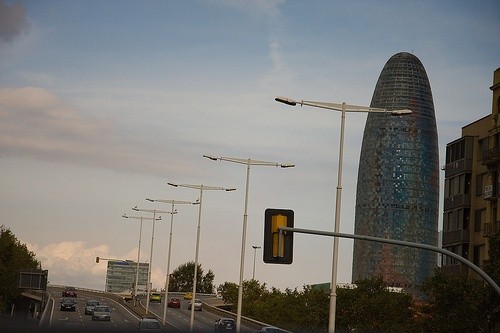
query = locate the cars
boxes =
[138,315,161,330]
[167,297,181,309]
[150,292,161,303]
[214,318,237,333]
[60,298,77,312]
[260,326,281,333]
[84,300,101,315]
[62,287,78,298]
[187,298,202,311]
[183,291,193,300]
[92,305,113,322]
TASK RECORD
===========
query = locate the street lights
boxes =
[274,96,414,333]
[146,197,201,328]
[131,206,179,314]
[202,153,296,333]
[166,181,237,333]
[121,214,163,307]
